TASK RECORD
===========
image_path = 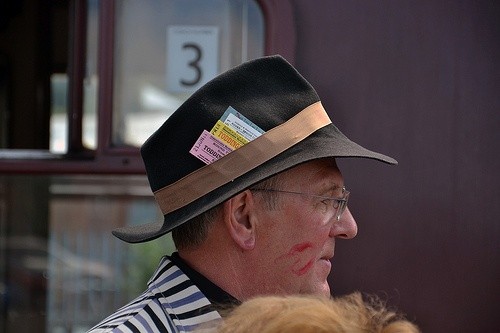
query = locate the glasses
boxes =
[249,185,350,222]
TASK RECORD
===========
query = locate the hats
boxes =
[111,54,398,243]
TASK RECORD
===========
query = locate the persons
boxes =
[81,54,398,333]
